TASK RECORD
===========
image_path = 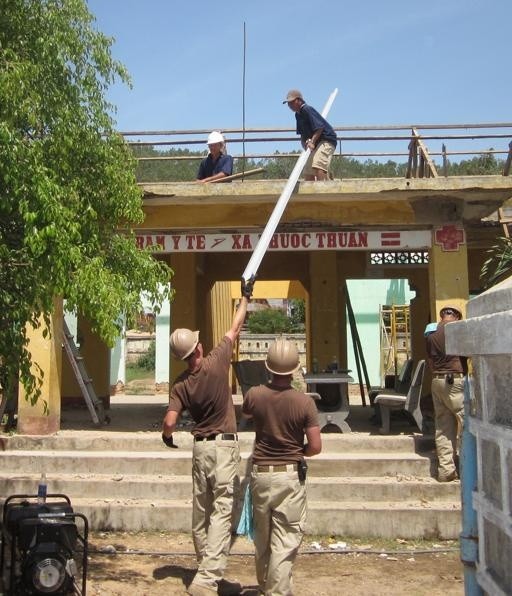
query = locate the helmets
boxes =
[440,304,462,321]
[206,131,225,145]
[170,328,201,360]
[265,339,300,376]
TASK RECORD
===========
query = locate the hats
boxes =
[282,89,302,103]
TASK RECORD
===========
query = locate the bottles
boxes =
[312,355,319,375]
[37,473,48,505]
[331,355,339,375]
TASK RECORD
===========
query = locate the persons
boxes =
[160,273,259,595]
[280,89,337,180]
[425,305,469,483]
[194,131,236,184]
[239,338,323,595]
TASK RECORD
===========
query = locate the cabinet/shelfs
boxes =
[378,303,412,389]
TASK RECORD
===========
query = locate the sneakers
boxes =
[186,579,243,596]
[438,471,457,481]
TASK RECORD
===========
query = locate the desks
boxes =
[304,372,354,434]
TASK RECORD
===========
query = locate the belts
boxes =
[258,464,299,472]
[433,373,461,379]
[195,434,238,440]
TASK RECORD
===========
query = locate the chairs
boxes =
[368,357,414,425]
[373,360,425,434]
[231,358,268,405]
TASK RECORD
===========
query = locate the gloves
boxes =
[240,277,255,300]
[162,433,178,448]
